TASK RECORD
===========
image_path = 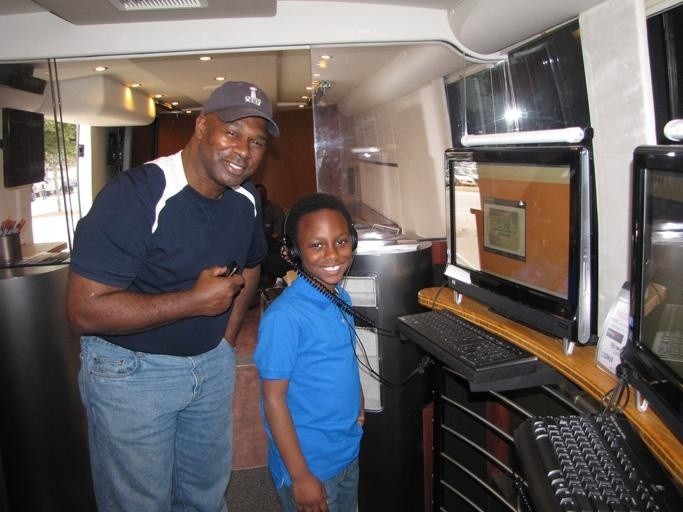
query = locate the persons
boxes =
[64,80,279,512]
[254,183,285,242]
[249,191,367,511]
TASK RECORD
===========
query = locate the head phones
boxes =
[280,193,358,261]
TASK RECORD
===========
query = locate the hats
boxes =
[201,81,280,139]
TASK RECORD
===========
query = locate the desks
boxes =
[416,284,683,511]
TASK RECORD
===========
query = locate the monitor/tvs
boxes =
[3,105,57,188]
[620,144,683,444]
[444,146,593,355]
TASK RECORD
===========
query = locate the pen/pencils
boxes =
[0,216,26,234]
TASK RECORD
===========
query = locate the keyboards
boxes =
[394,308,539,385]
[517,414,680,512]
